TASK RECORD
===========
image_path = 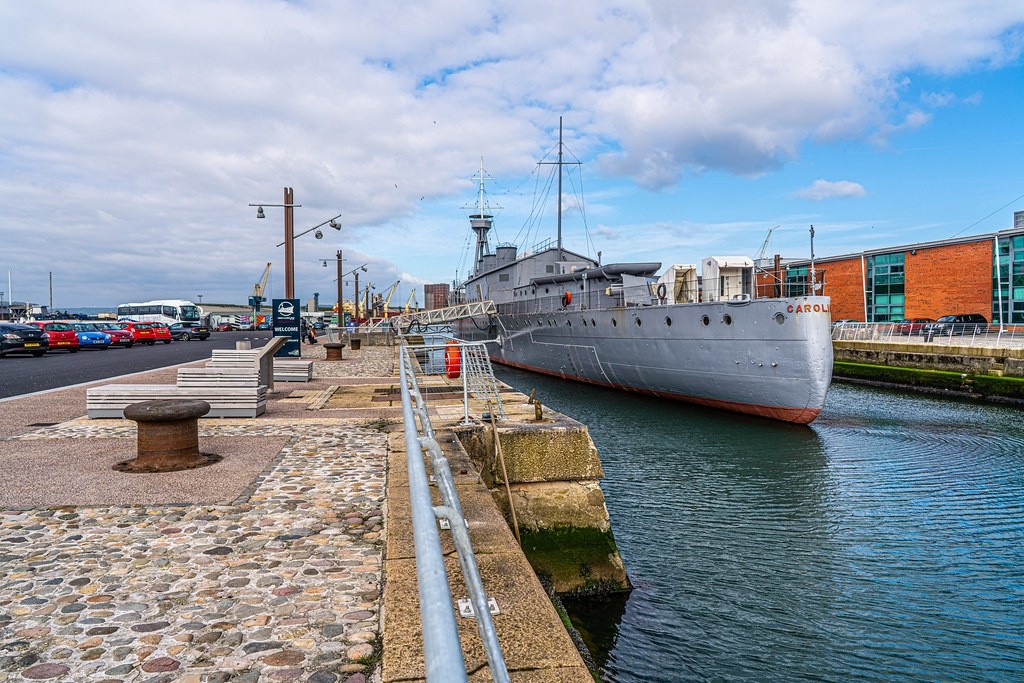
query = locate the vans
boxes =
[925,314,988,336]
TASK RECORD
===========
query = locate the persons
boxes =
[301,319,317,345]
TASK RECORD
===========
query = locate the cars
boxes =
[168,322,210,341]
[259,322,270,331]
[69,322,110,350]
[151,322,171,344]
[116,322,155,346]
[239,322,253,331]
[217,323,232,331]
[25,321,79,353]
[0,322,49,357]
[313,322,323,330]
[897,318,936,335]
[96,323,133,348]
[832,320,858,327]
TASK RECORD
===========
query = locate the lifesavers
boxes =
[562,296,567,307]
[657,282,667,299]
[443,340,461,379]
[564,291,572,305]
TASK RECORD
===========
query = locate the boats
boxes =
[454,116,834,424]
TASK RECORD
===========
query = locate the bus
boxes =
[118,300,203,329]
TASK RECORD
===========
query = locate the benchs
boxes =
[85,349,313,420]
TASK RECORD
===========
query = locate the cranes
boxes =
[249,263,271,312]
